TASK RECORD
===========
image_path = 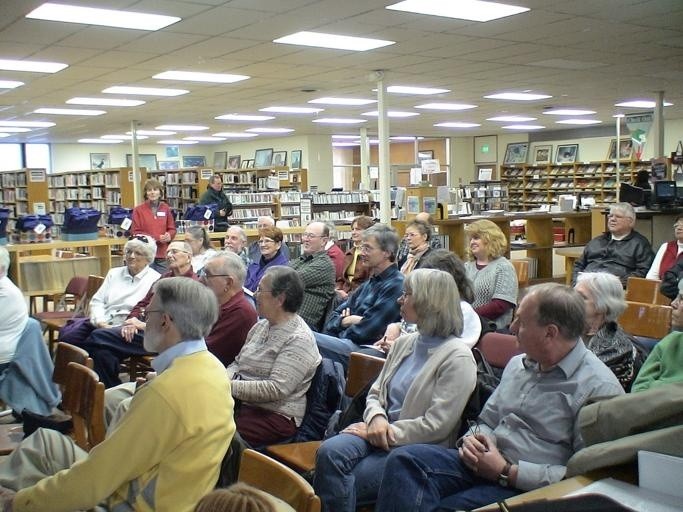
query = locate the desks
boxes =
[554,248,583,286]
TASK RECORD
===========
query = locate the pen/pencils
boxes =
[384,336,387,341]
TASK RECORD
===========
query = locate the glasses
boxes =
[201,270,229,280]
[128,236,149,243]
[138,306,173,322]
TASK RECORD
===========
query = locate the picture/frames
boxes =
[89,152,110,169]
[605,138,634,160]
[503,142,530,164]
[554,144,578,163]
[252,148,272,167]
[290,150,301,170]
[125,153,156,169]
[532,145,552,163]
[270,151,287,166]
[212,152,226,170]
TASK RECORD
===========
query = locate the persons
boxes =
[2,165,682,511]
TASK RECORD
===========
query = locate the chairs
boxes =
[478,332,522,373]
[267,350,387,475]
[118,355,157,383]
[237,447,320,511]
[619,275,673,340]
[28,275,104,356]
[51,342,108,451]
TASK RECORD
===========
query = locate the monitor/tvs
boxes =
[655,181,677,206]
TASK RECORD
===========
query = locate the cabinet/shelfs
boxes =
[0,158,671,242]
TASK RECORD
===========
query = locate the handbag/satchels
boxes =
[184,202,218,221]
[16,215,53,232]
[108,208,131,224]
[0,209,10,238]
[64,207,101,233]
[671,140,683,181]
